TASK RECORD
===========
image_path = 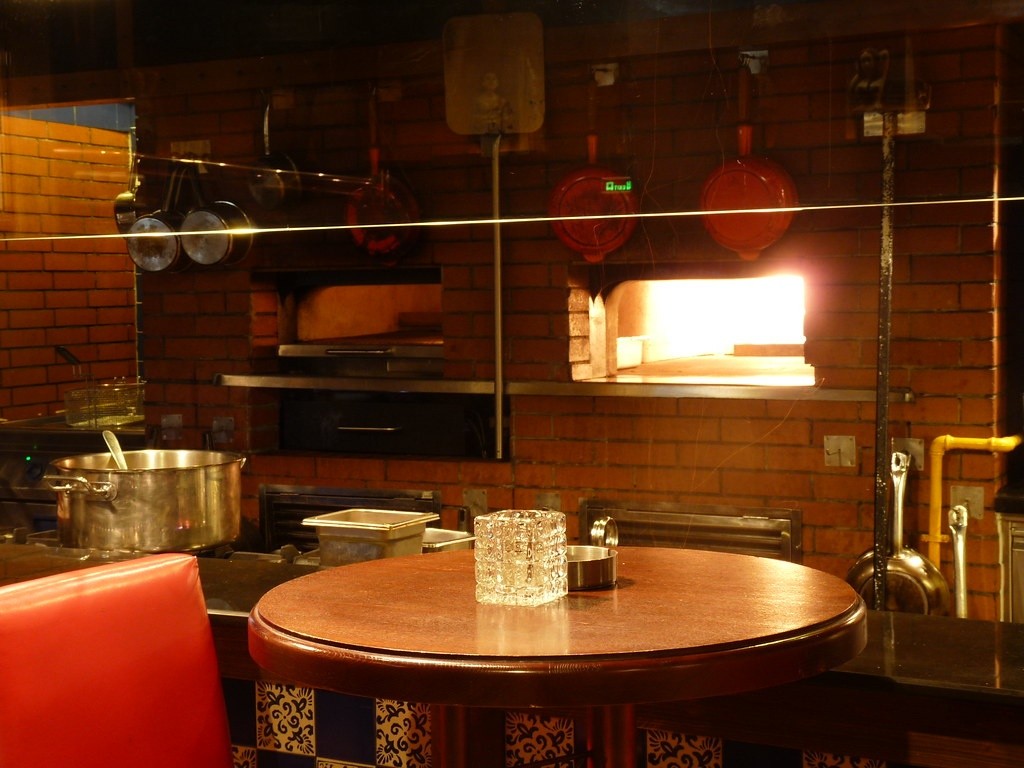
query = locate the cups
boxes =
[474,510,568,608]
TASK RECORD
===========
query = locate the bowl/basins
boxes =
[566,545,618,592]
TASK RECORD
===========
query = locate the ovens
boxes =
[0,451,94,533]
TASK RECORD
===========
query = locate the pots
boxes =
[114,151,151,241]
[247,102,303,210]
[698,66,798,262]
[126,153,186,272]
[843,450,951,617]
[44,449,246,553]
[342,88,424,266]
[179,152,253,268]
[547,83,639,262]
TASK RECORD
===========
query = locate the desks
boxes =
[248,544,869,768]
